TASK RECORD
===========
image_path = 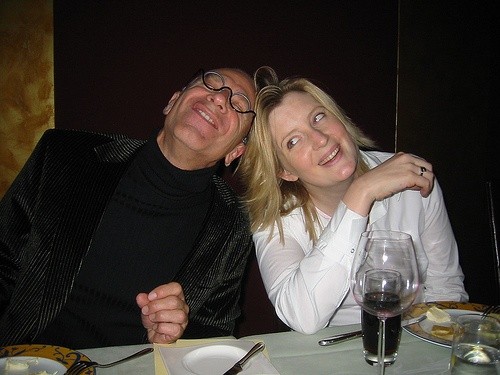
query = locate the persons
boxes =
[0,64,261,351]
[237,79,470,334]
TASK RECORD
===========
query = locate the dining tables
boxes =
[76,324,452,374]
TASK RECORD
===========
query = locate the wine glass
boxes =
[350,230,420,375]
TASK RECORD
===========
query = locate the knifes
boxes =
[224,341,266,375]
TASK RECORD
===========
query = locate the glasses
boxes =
[184,68,256,142]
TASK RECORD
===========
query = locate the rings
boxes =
[419,166,426,176]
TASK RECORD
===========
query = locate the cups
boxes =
[360,268,401,368]
[452,314,499,375]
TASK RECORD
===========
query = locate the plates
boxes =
[0,345,96,375]
[397,301,498,348]
[182,346,281,375]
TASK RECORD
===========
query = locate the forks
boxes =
[317,314,426,346]
[64,347,154,375]
